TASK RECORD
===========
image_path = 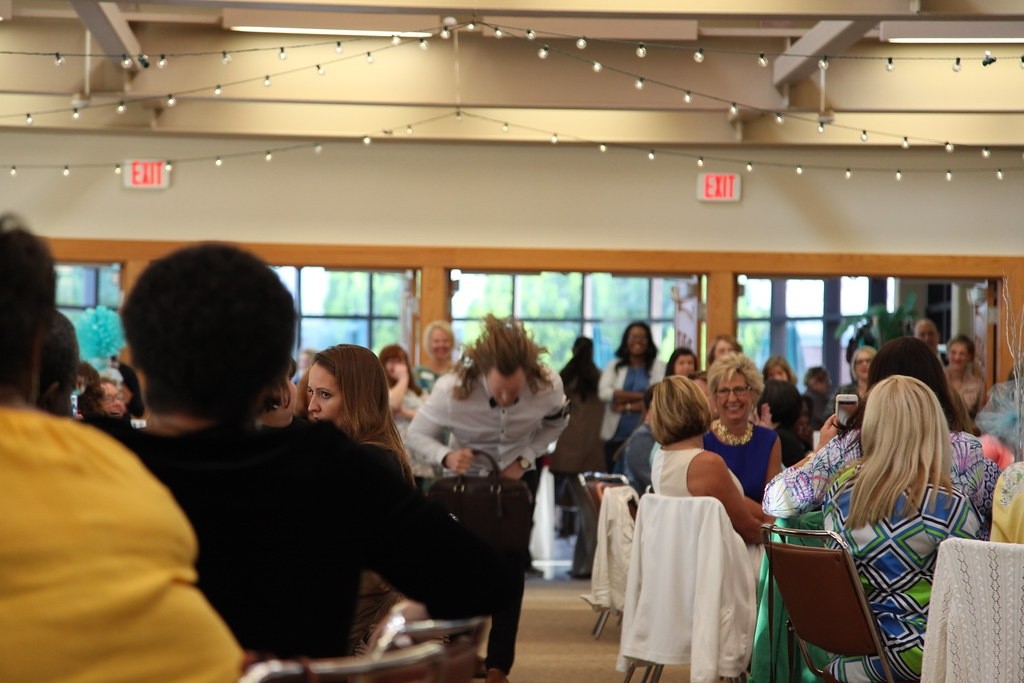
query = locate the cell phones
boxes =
[835,394,859,426]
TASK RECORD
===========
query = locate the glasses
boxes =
[627,335,649,344]
[102,392,124,404]
[716,386,751,398]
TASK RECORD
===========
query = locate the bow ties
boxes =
[490,396,519,408]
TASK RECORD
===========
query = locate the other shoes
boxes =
[523,566,543,578]
[474,659,487,676]
[485,670,509,683]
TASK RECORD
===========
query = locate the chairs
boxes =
[369,614,492,683]
[624,493,757,682]
[760,522,894,682]
[578,470,639,641]
[231,639,448,682]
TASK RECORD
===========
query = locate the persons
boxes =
[308,344,411,655]
[77,362,103,418]
[400,313,570,683]
[599,321,665,474]
[0,214,252,682]
[649,375,776,580]
[79,248,519,659]
[667,317,986,467]
[822,375,989,683]
[38,310,79,416]
[100,377,125,419]
[413,319,455,395]
[547,335,608,537]
[295,368,310,418]
[694,353,782,504]
[379,345,427,437]
[762,336,1001,542]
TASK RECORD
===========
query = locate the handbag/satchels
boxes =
[427,450,533,549]
